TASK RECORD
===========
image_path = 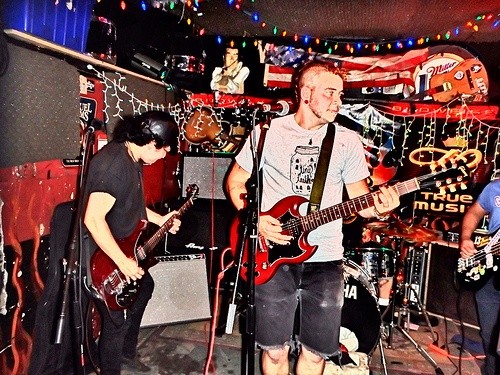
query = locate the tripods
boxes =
[368,239,445,375]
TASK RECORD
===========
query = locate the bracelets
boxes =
[374,212,392,221]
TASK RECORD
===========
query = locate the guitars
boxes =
[454,226,500,291]
[227,167,467,287]
[81,182,200,310]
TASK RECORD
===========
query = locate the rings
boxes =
[460,252,463,254]
[383,203,389,207]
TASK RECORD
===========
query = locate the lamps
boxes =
[130,52,169,81]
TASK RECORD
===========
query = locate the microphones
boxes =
[259,99,290,116]
[87,119,102,136]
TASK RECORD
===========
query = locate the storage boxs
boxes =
[1,0,93,53]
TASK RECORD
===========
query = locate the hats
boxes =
[138,111,184,161]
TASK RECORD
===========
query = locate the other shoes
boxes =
[120,354,152,375]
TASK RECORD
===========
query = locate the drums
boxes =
[341,258,384,357]
[342,247,400,278]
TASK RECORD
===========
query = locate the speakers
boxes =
[137,254,212,329]
[179,151,235,205]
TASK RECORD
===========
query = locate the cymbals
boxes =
[363,220,439,239]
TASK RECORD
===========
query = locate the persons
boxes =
[210,46,250,104]
[224,59,400,375]
[80,111,181,375]
[460,179,500,375]
